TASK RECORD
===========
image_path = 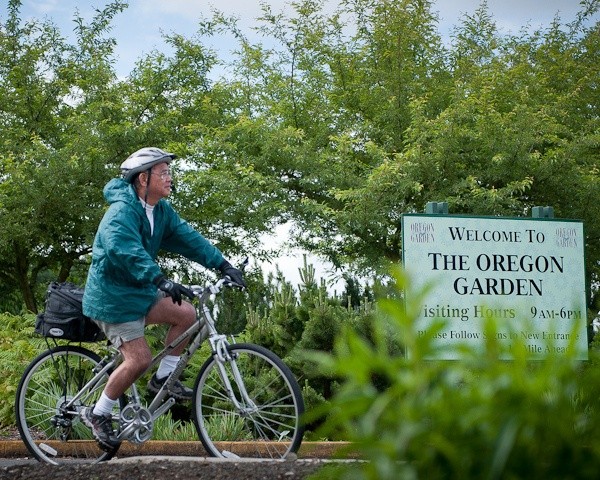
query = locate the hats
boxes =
[119,147,179,183]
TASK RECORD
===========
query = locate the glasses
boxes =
[147,169,174,179]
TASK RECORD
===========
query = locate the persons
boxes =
[80,146,245,452]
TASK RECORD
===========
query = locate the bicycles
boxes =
[15,257,305,466]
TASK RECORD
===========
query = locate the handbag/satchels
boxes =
[33,279,105,343]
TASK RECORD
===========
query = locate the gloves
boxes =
[156,275,196,306]
[219,260,250,290]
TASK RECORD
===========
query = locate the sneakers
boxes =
[81,407,122,456]
[146,373,197,402]
